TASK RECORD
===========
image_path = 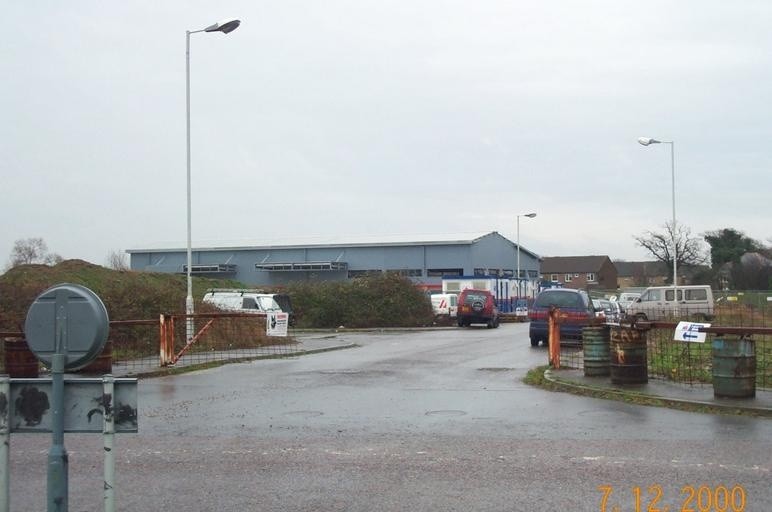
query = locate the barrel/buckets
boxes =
[581,325,610,378]
[712,336,756,399]
[609,323,649,384]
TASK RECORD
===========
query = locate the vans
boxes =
[430,294,457,326]
[202,288,295,326]
[625,284,715,323]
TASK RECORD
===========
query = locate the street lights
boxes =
[517,212,537,300]
[186,19,241,350]
[636,136,679,321]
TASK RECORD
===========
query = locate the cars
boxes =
[530,288,642,348]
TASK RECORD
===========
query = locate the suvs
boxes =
[457,289,501,329]
